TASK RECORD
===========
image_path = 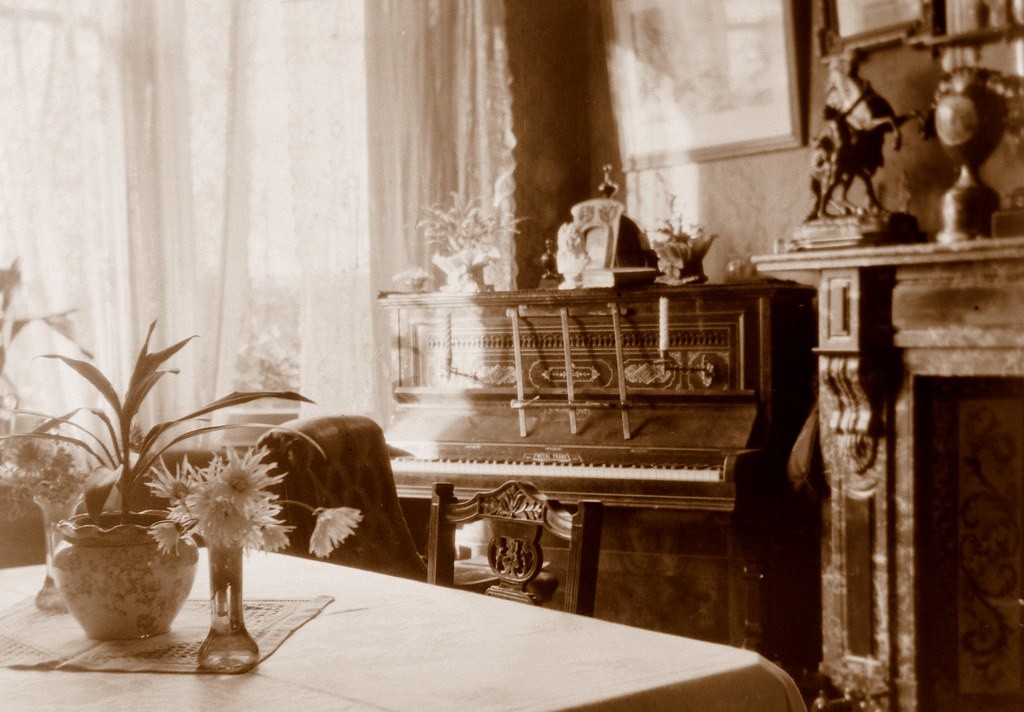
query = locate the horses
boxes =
[812,45,927,216]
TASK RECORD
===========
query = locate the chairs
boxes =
[427,482,603,616]
[252,416,432,583]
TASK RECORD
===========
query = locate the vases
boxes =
[197,548,259,672]
[32,495,86,611]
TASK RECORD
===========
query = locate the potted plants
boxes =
[1,320,327,641]
[413,180,528,294]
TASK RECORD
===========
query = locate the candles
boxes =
[657,297,671,351]
[442,314,452,359]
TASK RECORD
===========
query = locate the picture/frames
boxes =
[819,0,935,55]
[598,1,804,173]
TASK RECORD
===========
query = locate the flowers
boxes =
[144,438,364,560]
[1,424,145,522]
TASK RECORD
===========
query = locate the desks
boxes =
[2,549,808,712]
